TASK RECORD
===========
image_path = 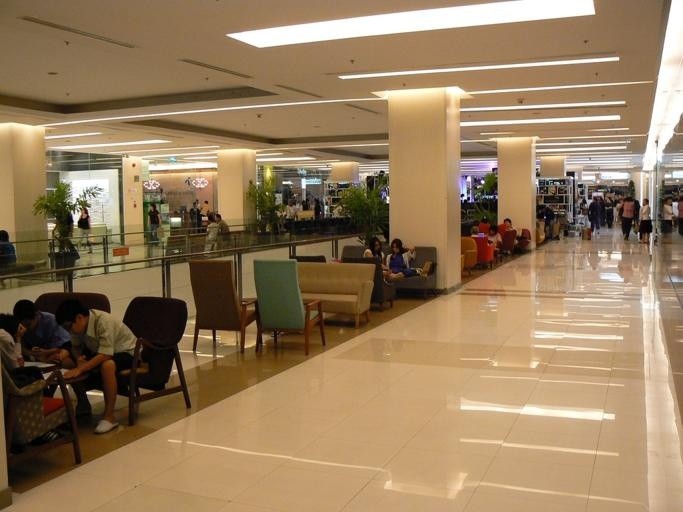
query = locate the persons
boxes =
[0,311,61,449]
[214,214,230,247]
[146,202,161,248]
[49,202,74,253]
[59,298,144,435]
[204,213,219,259]
[0,229,17,269]
[10,297,70,359]
[76,206,95,255]
[188,200,203,225]
[199,200,210,218]
[195,199,201,210]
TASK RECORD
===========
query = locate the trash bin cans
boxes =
[582,227,591,240]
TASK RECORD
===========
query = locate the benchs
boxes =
[341,245,438,299]
[295,262,376,328]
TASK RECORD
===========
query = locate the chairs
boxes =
[190,259,262,353]
[254,259,326,355]
[343,256,396,310]
[289,255,326,263]
[461,223,531,274]
[0,292,193,466]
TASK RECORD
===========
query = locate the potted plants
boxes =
[244,175,281,234]
[34,178,105,277]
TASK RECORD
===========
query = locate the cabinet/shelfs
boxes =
[534,174,573,225]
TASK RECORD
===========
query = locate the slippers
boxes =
[95,419,120,433]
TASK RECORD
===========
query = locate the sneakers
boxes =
[624,234,649,243]
